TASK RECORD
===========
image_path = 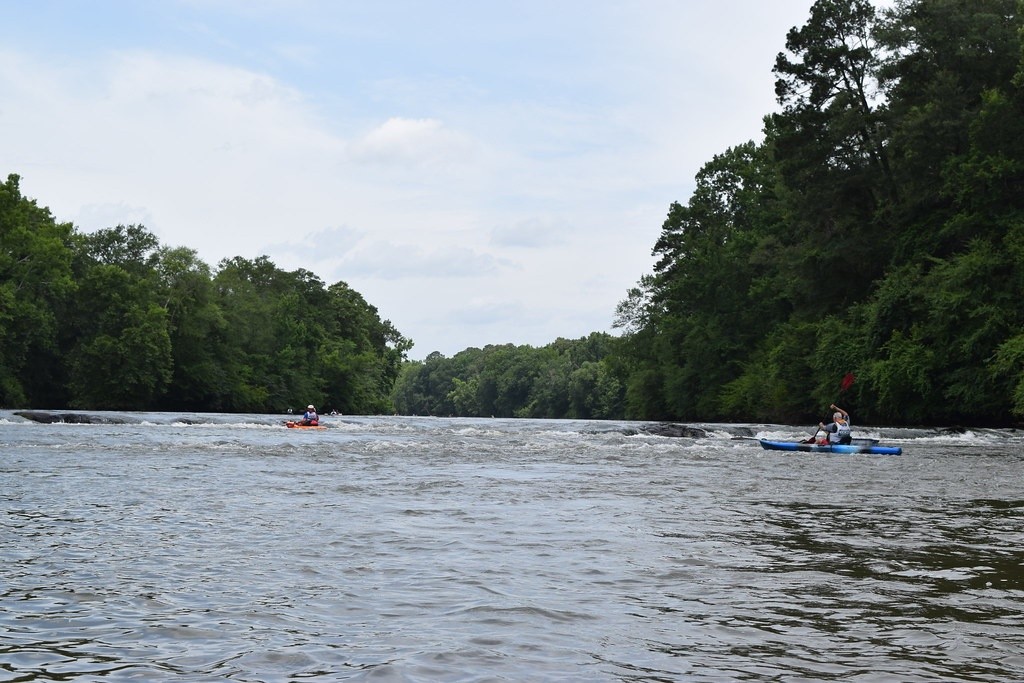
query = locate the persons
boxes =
[303,405,319,426]
[814,403,850,445]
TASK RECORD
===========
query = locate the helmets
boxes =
[308,405,314,408]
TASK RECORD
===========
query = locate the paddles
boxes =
[807,372,855,445]
